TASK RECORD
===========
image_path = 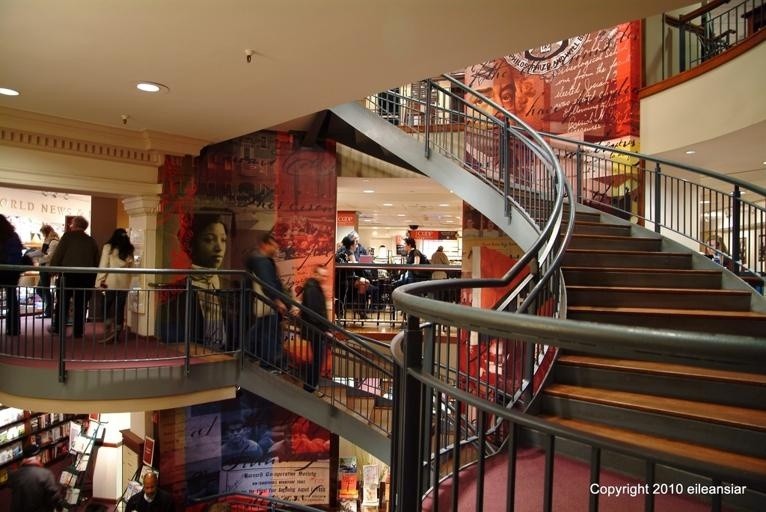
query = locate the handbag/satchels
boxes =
[20,253,35,273]
[254,280,274,317]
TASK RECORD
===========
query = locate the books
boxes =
[0,405,88,486]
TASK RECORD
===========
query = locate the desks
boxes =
[740,3,765,36]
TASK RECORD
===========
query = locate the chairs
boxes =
[335,256,374,328]
[377,268,417,328]
[694,25,737,61]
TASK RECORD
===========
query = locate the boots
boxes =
[96,319,121,344]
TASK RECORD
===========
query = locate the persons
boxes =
[158,210,241,344]
[241,234,290,374]
[347,230,381,280]
[3,443,73,512]
[124,471,175,512]
[401,236,423,283]
[703,235,729,263]
[297,279,336,390]
[266,423,332,461]
[465,59,528,184]
[33,225,59,320]
[483,220,500,238]
[333,236,380,320]
[0,214,26,336]
[218,419,266,465]
[92,227,135,344]
[45,215,101,340]
[463,218,480,238]
[428,245,451,280]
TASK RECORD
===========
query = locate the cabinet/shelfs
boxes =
[27,413,88,466]
[0,407,28,490]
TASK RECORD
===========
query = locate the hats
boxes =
[22,444,41,458]
